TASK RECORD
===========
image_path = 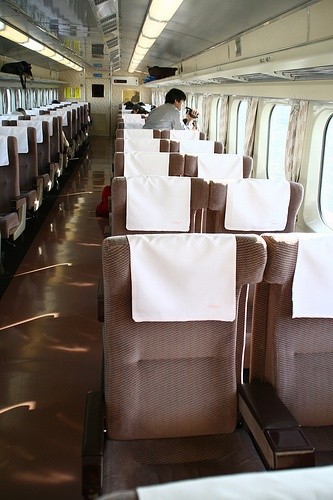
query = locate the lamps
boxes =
[127,0,183,74]
[0,21,84,72]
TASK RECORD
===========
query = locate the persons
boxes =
[142,88,197,130]
[16,108,26,115]
[126,103,134,109]
[52,100,60,104]
[133,104,149,114]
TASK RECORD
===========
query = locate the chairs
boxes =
[0,105,332,500]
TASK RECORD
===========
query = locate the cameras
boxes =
[186,106,199,117]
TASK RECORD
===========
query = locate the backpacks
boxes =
[146,65,178,77]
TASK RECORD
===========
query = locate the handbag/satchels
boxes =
[1,61,33,89]
